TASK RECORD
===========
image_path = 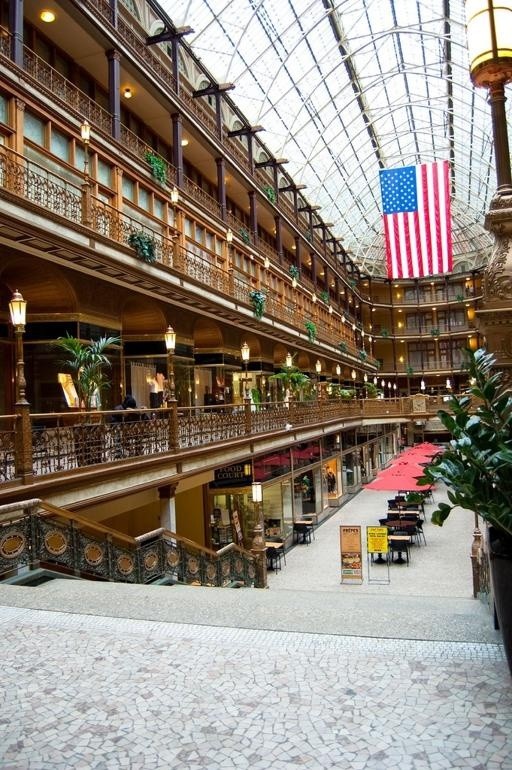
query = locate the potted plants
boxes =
[46,329,123,468]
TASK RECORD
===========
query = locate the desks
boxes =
[266,542,286,566]
[295,521,315,542]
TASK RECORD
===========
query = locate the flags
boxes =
[379,160,451,279]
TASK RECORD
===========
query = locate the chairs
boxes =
[294,523,311,545]
[371,489,435,566]
[266,547,282,571]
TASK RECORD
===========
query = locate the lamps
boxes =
[125,88,131,98]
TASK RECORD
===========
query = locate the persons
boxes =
[204,386,213,413]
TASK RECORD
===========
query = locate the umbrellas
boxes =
[254,446,329,480]
[362,441,446,523]
